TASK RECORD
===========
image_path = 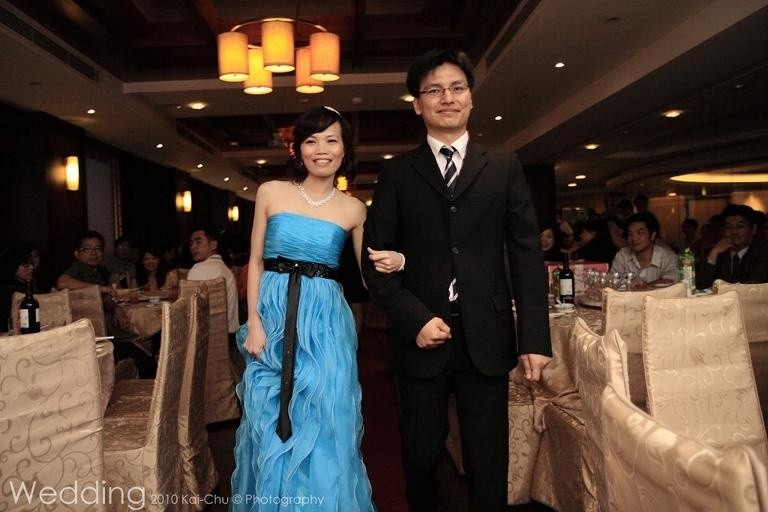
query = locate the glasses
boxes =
[420,85,470,98]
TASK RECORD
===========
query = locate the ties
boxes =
[439,145,459,197]
[733,253,739,275]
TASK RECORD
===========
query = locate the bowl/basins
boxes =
[548,293,575,312]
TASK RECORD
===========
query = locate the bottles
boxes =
[678,243,697,294]
[19,278,41,335]
[559,250,575,305]
[552,267,561,295]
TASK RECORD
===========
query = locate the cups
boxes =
[7,315,66,337]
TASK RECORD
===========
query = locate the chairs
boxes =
[0,253,240,510]
[474,266,767,512]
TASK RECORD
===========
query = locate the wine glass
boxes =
[110,273,120,298]
[581,267,635,302]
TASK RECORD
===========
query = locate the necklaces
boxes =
[298,183,336,208]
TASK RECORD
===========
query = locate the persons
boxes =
[0,222,247,379]
[360,45,556,512]
[228,104,408,512]
[539,203,767,292]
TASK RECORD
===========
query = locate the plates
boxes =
[106,288,177,303]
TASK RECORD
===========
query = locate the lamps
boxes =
[66,156,79,191]
[184,191,192,213]
[214,11,345,96]
[233,206,239,221]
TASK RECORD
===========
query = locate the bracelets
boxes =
[396,253,406,272]
